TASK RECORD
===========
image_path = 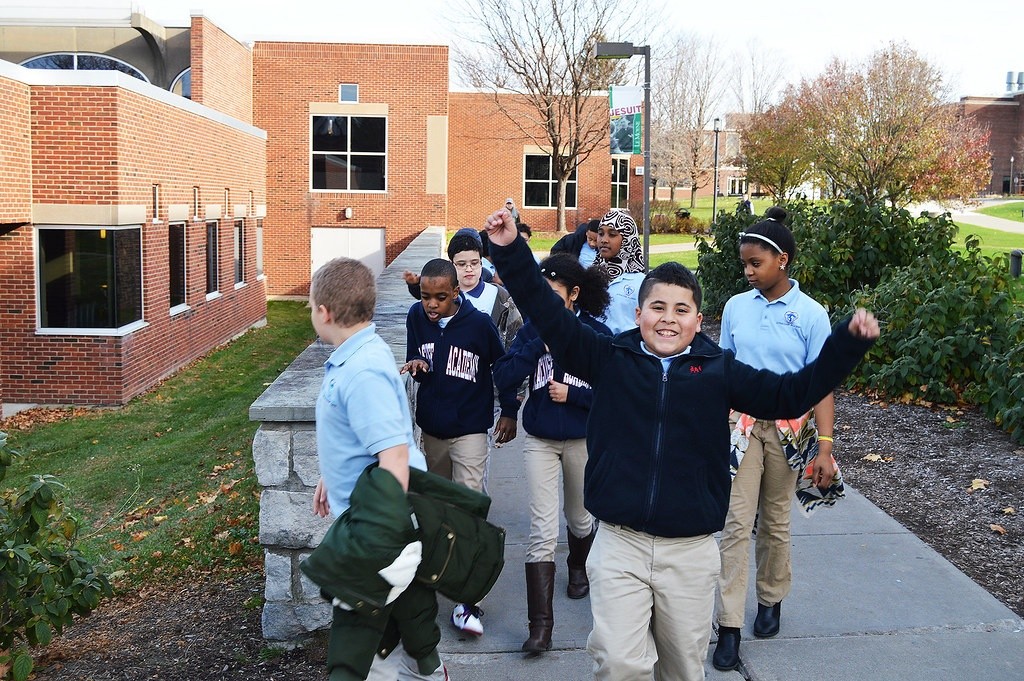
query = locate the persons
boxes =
[713,207,835,671]
[404,222,541,496]
[584,210,648,335]
[493,253,613,653]
[550,220,601,269]
[737,193,754,215]
[401,259,521,635]
[308,257,450,681]
[503,197,520,223]
[484,210,880,681]
[610,117,633,152]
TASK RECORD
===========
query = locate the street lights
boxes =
[592,41,653,275]
[710,117,725,224]
[989,157,996,195]
[1008,156,1015,195]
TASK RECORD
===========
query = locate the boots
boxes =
[567,523,594,599]
[522,561,557,652]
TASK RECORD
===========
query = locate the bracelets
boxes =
[818,435,833,442]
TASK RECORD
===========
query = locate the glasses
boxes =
[451,259,483,270]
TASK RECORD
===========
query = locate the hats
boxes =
[506,198,513,204]
[456,227,484,249]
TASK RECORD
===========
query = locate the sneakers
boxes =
[450,603,484,636]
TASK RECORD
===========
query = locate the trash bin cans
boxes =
[675,208,690,220]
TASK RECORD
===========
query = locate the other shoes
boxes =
[396,653,450,681]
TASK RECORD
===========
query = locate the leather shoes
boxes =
[754,600,782,637]
[712,629,741,671]
